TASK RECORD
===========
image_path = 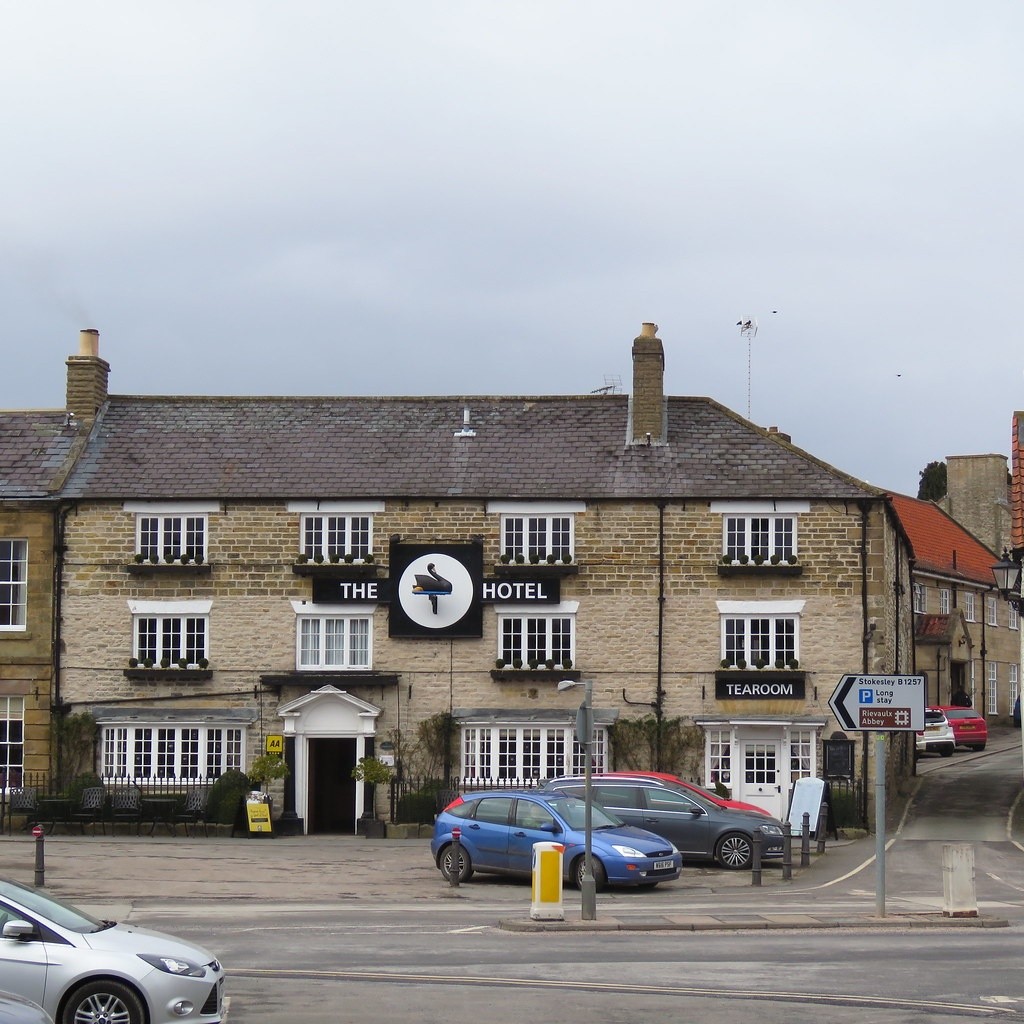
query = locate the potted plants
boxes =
[351,755,394,839]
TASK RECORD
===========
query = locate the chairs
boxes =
[644,789,652,809]
[70,787,106,836]
[111,787,142,837]
[7,787,40,836]
[174,789,208,838]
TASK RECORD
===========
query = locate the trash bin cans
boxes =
[528,841,566,920]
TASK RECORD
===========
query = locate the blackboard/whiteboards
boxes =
[786,777,824,832]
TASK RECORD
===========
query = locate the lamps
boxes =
[988,545,1024,618]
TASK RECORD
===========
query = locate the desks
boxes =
[40,798,78,836]
[141,799,178,837]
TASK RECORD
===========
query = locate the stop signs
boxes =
[32,827,43,837]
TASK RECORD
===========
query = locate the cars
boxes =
[915,708,956,757]
[431,787,684,893]
[0,875,226,1023]
[576,773,773,818]
[929,705,988,752]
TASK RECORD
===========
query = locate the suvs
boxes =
[538,774,786,871]
